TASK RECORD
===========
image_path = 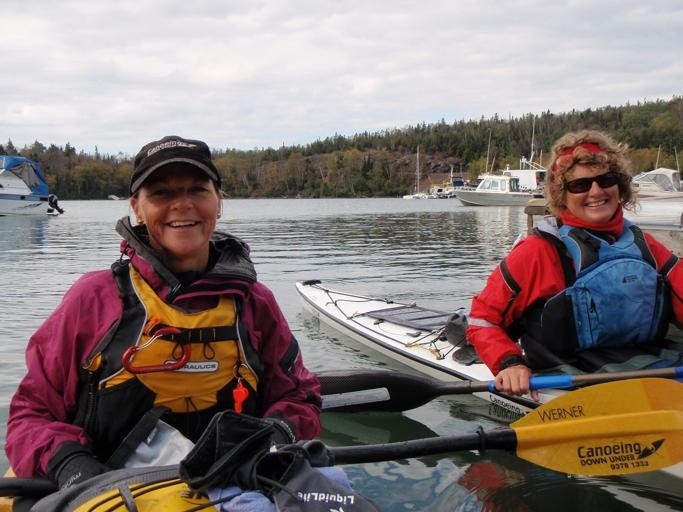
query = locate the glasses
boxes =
[566,172,620,193]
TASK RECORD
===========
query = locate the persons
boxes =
[7,132,386,510]
[465,126,682,408]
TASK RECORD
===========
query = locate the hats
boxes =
[130,135,221,197]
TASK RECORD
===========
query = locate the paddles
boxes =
[313,366,683,414]
[0,377,682,497]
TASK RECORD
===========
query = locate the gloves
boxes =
[261,416,296,448]
[48,443,114,491]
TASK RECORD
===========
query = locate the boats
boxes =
[402,114,683,232]
[294,278,683,498]
[0,154,65,217]
